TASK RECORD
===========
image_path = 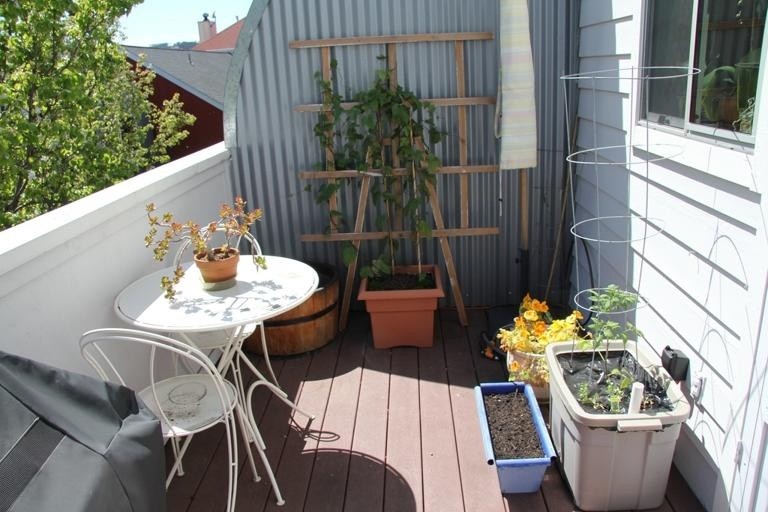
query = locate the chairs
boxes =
[76,327,284,511]
[173,224,317,480]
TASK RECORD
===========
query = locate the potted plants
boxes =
[495,293,587,402]
[139,195,270,306]
[307,63,446,349]
[543,282,690,512]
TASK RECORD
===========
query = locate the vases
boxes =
[472,380,558,497]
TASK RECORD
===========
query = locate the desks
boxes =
[113,252,323,512]
